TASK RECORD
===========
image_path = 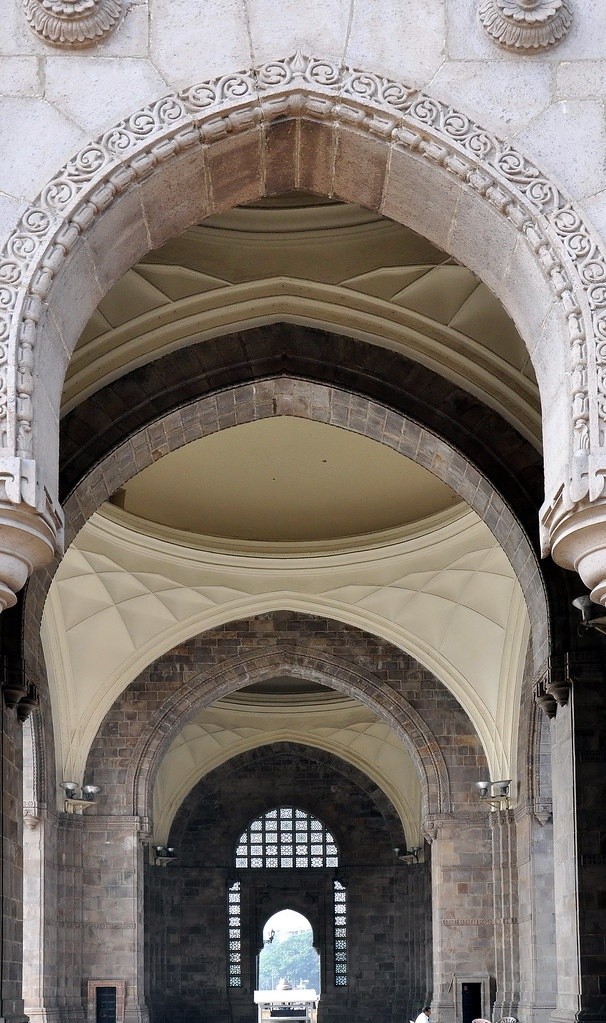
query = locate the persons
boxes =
[415,1006,431,1023]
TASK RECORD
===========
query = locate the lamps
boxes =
[151,845,177,865]
[60,779,100,813]
[571,595,594,622]
[474,778,513,803]
[395,846,422,865]
[264,928,275,943]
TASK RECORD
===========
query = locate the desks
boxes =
[253,988,317,1023]
